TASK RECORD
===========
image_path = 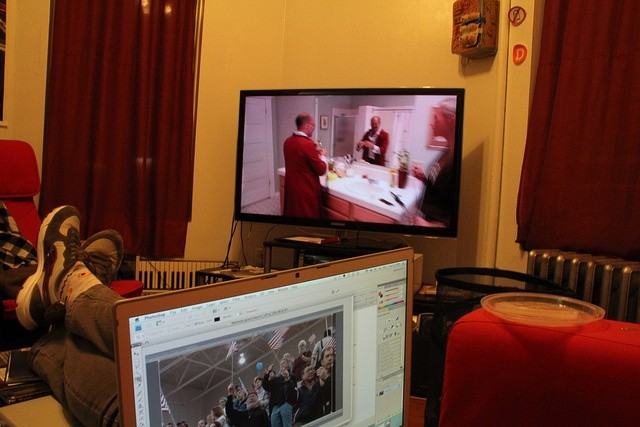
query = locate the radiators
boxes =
[526,248,640,326]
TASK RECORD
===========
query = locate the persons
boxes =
[16,205,126,427]
[283,111,326,218]
[407,97,456,227]
[356,115,390,166]
[166,329,336,427]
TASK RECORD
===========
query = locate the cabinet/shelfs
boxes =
[197,264,486,427]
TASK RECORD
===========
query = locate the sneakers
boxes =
[14,203,82,332]
[82,227,124,285]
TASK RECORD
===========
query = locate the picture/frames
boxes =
[320,113,329,130]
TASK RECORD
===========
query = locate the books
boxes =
[0,347,53,404]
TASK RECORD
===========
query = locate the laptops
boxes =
[112,246,414,427]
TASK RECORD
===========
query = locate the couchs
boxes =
[444,309,640,427]
[0,140,144,352]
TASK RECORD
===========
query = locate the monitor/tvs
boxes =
[236,88,464,238]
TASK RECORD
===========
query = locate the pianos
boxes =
[134,255,237,294]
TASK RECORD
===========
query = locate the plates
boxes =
[481,292,606,328]
